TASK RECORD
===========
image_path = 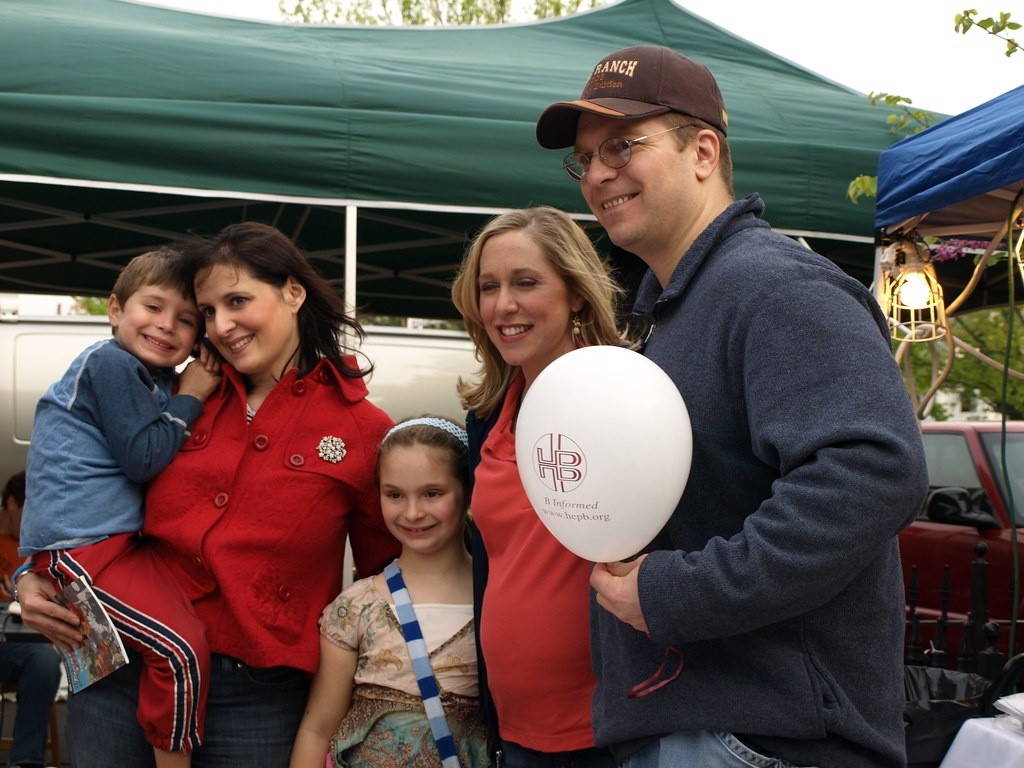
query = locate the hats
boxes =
[536,46,728,150]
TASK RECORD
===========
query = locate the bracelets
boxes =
[13,570,33,603]
[10,564,34,602]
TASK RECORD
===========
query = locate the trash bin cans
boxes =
[903,664,996,768]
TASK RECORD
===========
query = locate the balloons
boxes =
[513,343,693,567]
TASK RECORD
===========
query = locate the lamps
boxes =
[887,239,947,343]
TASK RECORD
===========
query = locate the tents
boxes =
[873,84,1024,325]
[3,0,1024,364]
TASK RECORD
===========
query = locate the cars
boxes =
[898,420,1024,677]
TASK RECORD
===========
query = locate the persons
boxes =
[0,222,402,768]
[287,414,497,768]
[533,44,932,768]
[455,204,642,768]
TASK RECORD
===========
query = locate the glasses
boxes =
[2,493,25,510]
[561,123,706,182]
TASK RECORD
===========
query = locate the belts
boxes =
[0,632,53,643]
